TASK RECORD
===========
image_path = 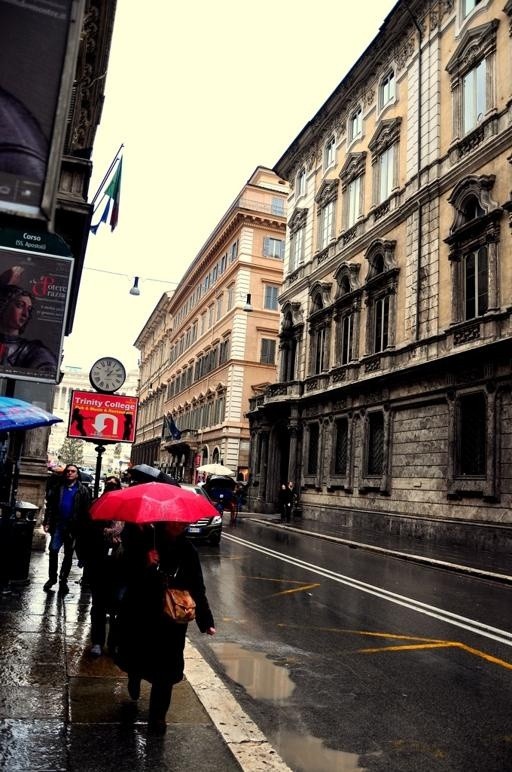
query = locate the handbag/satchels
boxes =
[162,589,196,623]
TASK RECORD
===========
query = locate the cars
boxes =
[178,482,222,544]
[80,466,95,474]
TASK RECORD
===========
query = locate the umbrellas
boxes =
[0,394,66,433]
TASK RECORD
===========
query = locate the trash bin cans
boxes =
[7,501,39,586]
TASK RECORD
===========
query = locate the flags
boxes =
[104,155,123,230]
[164,412,183,440]
[167,414,182,437]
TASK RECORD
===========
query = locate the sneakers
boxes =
[148,712,166,731]
[43,579,117,657]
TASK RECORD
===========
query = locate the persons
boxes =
[202,478,214,500]
[111,521,215,735]
[43,464,153,655]
[171,474,187,483]
[231,485,241,520]
[285,481,297,524]
[0,284,58,375]
[277,484,287,522]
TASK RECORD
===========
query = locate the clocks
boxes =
[89,356,126,393]
